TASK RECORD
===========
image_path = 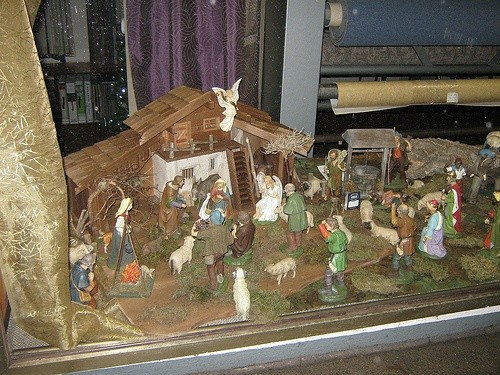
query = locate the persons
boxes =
[68,127,500,309]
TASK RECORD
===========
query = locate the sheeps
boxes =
[169,236,195,275]
[140,265,155,279]
[99,224,132,254]
[142,234,168,259]
[232,267,250,318]
[302,175,446,245]
[274,203,314,234]
[264,257,296,285]
